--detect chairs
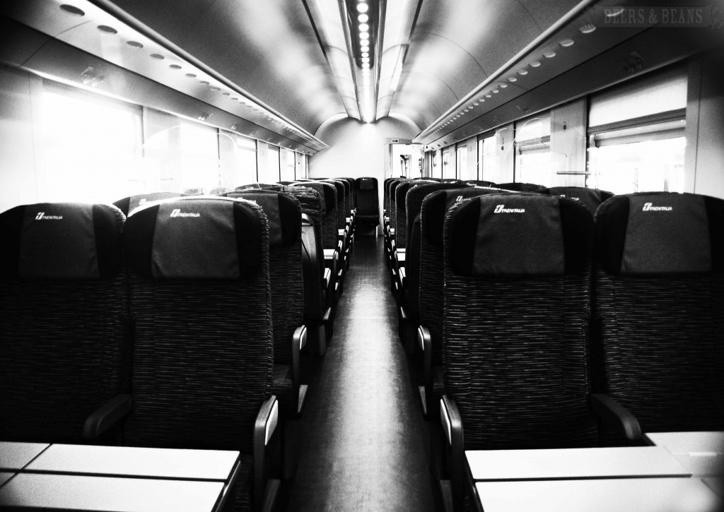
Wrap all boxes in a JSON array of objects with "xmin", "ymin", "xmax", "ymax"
[
  {"xmin": 591, "ymin": 190, "xmax": 724, "ymax": 443},
  {"xmin": 381, "ymin": 176, "xmax": 724, "ymax": 511},
  {"xmin": 433, "ymin": 190, "xmax": 648, "ymax": 511},
  {"xmin": 82, "ymin": 199, "xmax": 284, "ymax": 511},
  {"xmin": 1, "ymin": 202, "xmax": 130, "ymax": 446},
  {"xmin": 0, "ymin": 176, "xmax": 379, "ymax": 512}
]
